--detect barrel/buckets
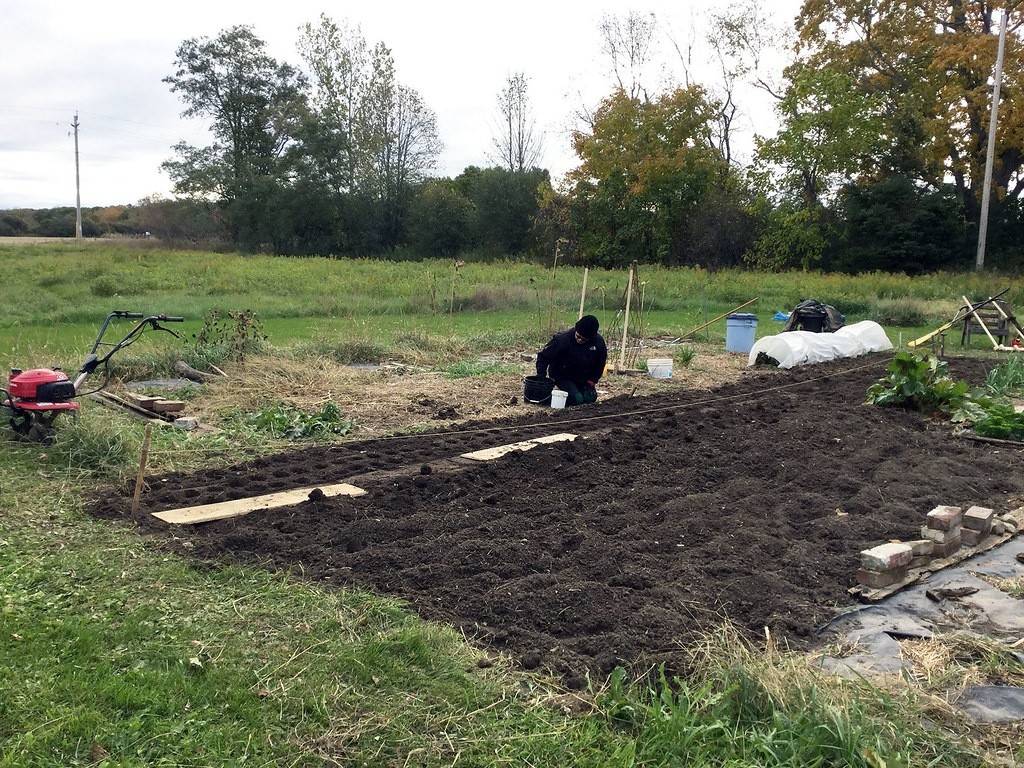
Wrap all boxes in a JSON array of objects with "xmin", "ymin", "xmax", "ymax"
[
  {"xmin": 726, "ymin": 312, "xmax": 759, "ymax": 353},
  {"xmin": 550, "ymin": 390, "xmax": 568, "ymax": 409},
  {"xmin": 521, "ymin": 376, "xmax": 555, "ymax": 407},
  {"xmin": 647, "ymin": 359, "xmax": 673, "ymax": 379}
]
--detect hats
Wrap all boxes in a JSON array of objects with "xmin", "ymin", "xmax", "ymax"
[{"xmin": 575, "ymin": 315, "xmax": 599, "ymax": 338}]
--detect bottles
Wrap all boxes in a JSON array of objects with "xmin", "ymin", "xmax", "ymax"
[{"xmin": 1010, "ymin": 338, "xmax": 1021, "ymax": 348}]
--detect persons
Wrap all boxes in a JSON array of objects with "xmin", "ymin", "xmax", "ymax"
[{"xmin": 536, "ymin": 315, "xmax": 607, "ymax": 406}]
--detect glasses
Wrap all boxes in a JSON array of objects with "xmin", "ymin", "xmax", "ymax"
[{"xmin": 575, "ymin": 333, "xmax": 587, "ymax": 342}]
